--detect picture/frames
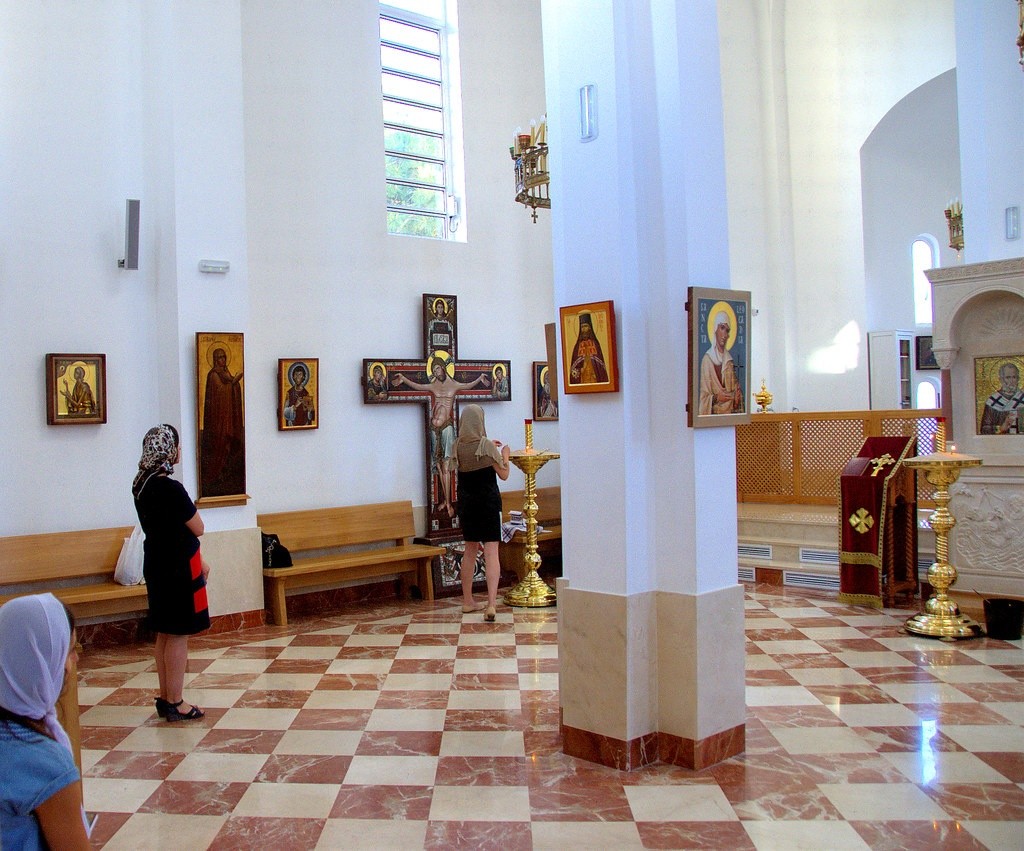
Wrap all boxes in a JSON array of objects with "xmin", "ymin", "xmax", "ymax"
[
  {"xmin": 46, "ymin": 354, "xmax": 107, "ymax": 425},
  {"xmin": 195, "ymin": 332, "xmax": 252, "ymax": 503},
  {"xmin": 916, "ymin": 336, "xmax": 940, "ymax": 370},
  {"xmin": 688, "ymin": 286, "xmax": 751, "ymax": 428},
  {"xmin": 533, "ymin": 361, "xmax": 559, "ymax": 421},
  {"xmin": 278, "ymin": 358, "xmax": 321, "ymax": 431},
  {"xmin": 559, "ymin": 300, "xmax": 619, "ymax": 393}
]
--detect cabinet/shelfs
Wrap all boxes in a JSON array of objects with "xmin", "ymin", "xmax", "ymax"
[{"xmin": 868, "ymin": 329, "xmax": 915, "ymax": 409}]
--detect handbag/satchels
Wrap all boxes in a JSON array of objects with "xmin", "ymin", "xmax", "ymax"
[
  {"xmin": 113, "ymin": 523, "xmax": 146, "ymax": 586},
  {"xmin": 261, "ymin": 531, "xmax": 293, "ymax": 568}
]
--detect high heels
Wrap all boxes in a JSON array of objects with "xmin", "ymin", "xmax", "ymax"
[{"xmin": 165, "ymin": 700, "xmax": 205, "ymax": 722}]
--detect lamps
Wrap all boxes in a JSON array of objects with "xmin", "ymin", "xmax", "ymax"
[{"xmin": 510, "ymin": 112, "xmax": 552, "ymax": 223}]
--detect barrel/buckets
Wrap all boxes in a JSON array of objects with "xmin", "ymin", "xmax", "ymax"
[{"xmin": 982, "ymin": 598, "xmax": 1024, "ymax": 640}]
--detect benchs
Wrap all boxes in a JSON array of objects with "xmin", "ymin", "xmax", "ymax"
[
  {"xmin": 257, "ymin": 501, "xmax": 446, "ymax": 625},
  {"xmin": 498, "ymin": 486, "xmax": 562, "ymax": 583},
  {"xmin": 0, "ymin": 526, "xmax": 149, "ymax": 634}
]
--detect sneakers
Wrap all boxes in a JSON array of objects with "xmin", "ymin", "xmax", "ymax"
[
  {"xmin": 483, "ymin": 606, "xmax": 496, "ymax": 621},
  {"xmin": 462, "ymin": 601, "xmax": 488, "ymax": 612}
]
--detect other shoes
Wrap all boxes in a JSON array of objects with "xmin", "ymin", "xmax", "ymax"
[{"xmin": 154, "ymin": 697, "xmax": 169, "ymax": 718}]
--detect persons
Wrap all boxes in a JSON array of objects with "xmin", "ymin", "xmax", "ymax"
[
  {"xmin": 450, "ymin": 404, "xmax": 511, "ymax": 622},
  {"xmin": 0, "ymin": 591, "xmax": 94, "ymax": 851},
  {"xmin": 132, "ymin": 423, "xmax": 211, "ymax": 722}
]
